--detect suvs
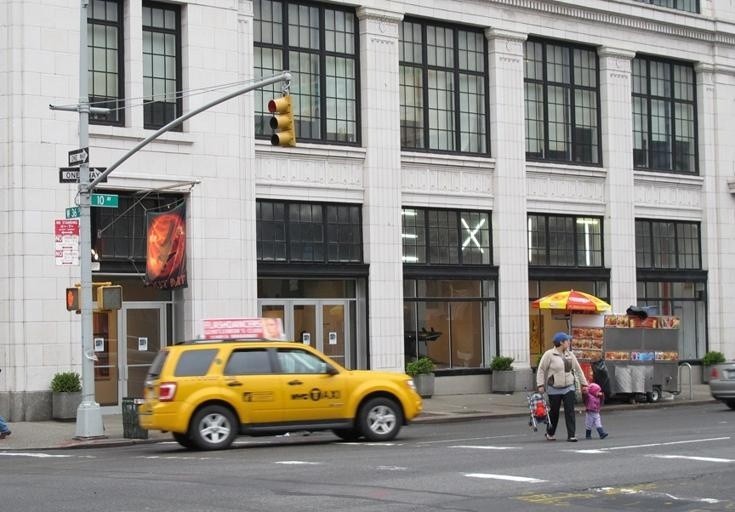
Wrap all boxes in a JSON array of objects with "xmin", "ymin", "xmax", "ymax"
[{"xmin": 137, "ymin": 336, "xmax": 423, "ymax": 452}]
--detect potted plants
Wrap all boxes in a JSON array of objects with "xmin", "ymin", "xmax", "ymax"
[
  {"xmin": 405, "ymin": 356, "xmax": 435, "ymax": 397},
  {"xmin": 702, "ymin": 351, "xmax": 725, "ymax": 382},
  {"xmin": 51, "ymin": 372, "xmax": 83, "ymax": 420},
  {"xmin": 490, "ymin": 354, "xmax": 516, "ymax": 394}
]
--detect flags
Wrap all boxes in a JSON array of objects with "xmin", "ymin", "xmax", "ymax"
[{"xmin": 145, "ymin": 204, "xmax": 189, "ymax": 290}]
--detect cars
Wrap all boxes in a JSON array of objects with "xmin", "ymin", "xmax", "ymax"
[{"xmin": 710, "ymin": 359, "xmax": 735, "ymax": 410}]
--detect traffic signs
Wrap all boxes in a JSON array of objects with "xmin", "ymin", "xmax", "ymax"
[
  {"xmin": 67, "ymin": 148, "xmax": 88, "ymax": 165},
  {"xmin": 59, "ymin": 168, "xmax": 108, "ymax": 183}
]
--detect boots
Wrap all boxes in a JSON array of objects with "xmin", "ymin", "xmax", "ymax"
[
  {"xmin": 597, "ymin": 427, "xmax": 609, "ymax": 439},
  {"xmin": 586, "ymin": 430, "xmax": 592, "ymax": 440}
]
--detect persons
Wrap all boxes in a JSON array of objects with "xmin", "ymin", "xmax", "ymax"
[
  {"xmin": 583, "ymin": 383, "xmax": 609, "ymax": 440},
  {"xmin": 536, "ymin": 332, "xmax": 590, "ymax": 442}
]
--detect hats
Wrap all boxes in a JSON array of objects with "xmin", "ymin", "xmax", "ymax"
[{"xmin": 552, "ymin": 331, "xmax": 573, "ymax": 343}]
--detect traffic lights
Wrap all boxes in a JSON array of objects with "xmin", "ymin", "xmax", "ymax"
[
  {"xmin": 268, "ymin": 96, "xmax": 295, "ymax": 147},
  {"xmin": 97, "ymin": 283, "xmax": 123, "ymax": 312},
  {"xmin": 66, "ymin": 287, "xmax": 80, "ymax": 312}
]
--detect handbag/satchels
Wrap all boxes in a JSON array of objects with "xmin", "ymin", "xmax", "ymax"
[{"xmin": 572, "ymin": 368, "xmax": 583, "ymax": 404}]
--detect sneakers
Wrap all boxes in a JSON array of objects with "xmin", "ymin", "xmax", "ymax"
[
  {"xmin": 545, "ymin": 433, "xmax": 557, "ymax": 440},
  {"xmin": 567, "ymin": 436, "xmax": 577, "ymax": 442}
]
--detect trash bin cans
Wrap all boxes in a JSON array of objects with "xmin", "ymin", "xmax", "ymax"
[{"xmin": 122, "ymin": 397, "xmax": 148, "ymax": 439}]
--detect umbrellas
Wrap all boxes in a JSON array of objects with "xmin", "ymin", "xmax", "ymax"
[{"xmin": 533, "ymin": 288, "xmax": 612, "ymax": 355}]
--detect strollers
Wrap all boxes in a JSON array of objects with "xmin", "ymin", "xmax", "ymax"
[{"xmin": 527, "ymin": 390, "xmax": 554, "ymax": 432}]
